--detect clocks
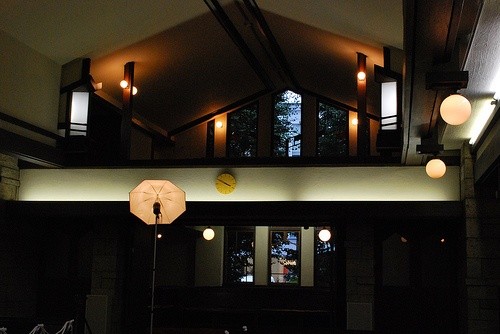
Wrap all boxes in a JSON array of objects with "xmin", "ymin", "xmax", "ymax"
[{"xmin": 214, "ymin": 171, "xmax": 236, "ymax": 195}]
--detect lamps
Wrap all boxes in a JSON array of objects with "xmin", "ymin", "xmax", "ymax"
[
  {"xmin": 423, "ymin": 68, "xmax": 473, "ymax": 126},
  {"xmin": 416, "ymin": 144, "xmax": 447, "ymax": 178}
]
